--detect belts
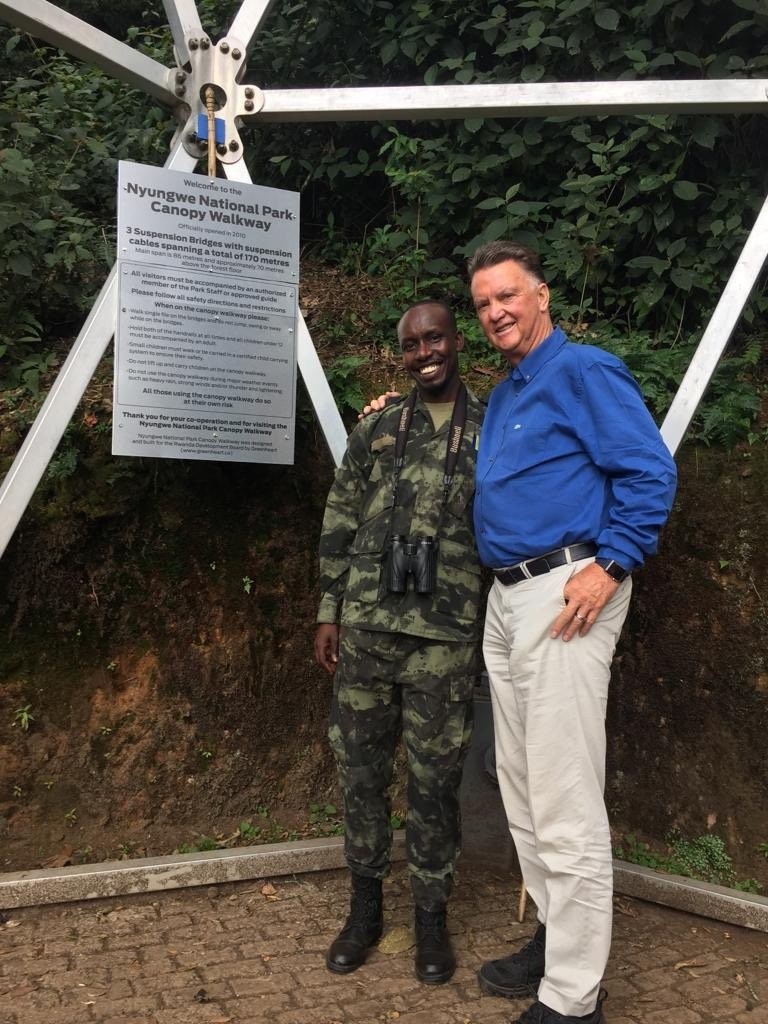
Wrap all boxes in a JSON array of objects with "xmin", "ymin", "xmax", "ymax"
[{"xmin": 488, "ymin": 542, "xmax": 599, "ymax": 585}]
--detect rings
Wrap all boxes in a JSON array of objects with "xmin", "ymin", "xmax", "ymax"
[{"xmin": 573, "ymin": 612, "xmax": 583, "ymax": 622}]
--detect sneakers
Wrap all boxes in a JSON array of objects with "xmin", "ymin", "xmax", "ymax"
[
  {"xmin": 475, "ymin": 923, "xmax": 545, "ymax": 998},
  {"xmin": 510, "ymin": 987, "xmax": 609, "ymax": 1024}
]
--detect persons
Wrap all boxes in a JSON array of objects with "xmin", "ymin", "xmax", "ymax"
[
  {"xmin": 349, "ymin": 233, "xmax": 679, "ymax": 1024},
  {"xmin": 314, "ymin": 293, "xmax": 494, "ymax": 981}
]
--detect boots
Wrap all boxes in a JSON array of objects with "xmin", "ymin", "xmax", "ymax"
[
  {"xmin": 325, "ymin": 896, "xmax": 383, "ymax": 974},
  {"xmin": 410, "ymin": 906, "xmax": 456, "ymax": 985}
]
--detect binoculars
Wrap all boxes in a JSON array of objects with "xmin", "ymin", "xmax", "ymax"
[{"xmin": 389, "ymin": 535, "xmax": 432, "ymax": 596}]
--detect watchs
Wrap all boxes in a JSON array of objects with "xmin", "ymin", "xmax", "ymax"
[{"xmin": 593, "ymin": 558, "xmax": 627, "ymax": 582}]
